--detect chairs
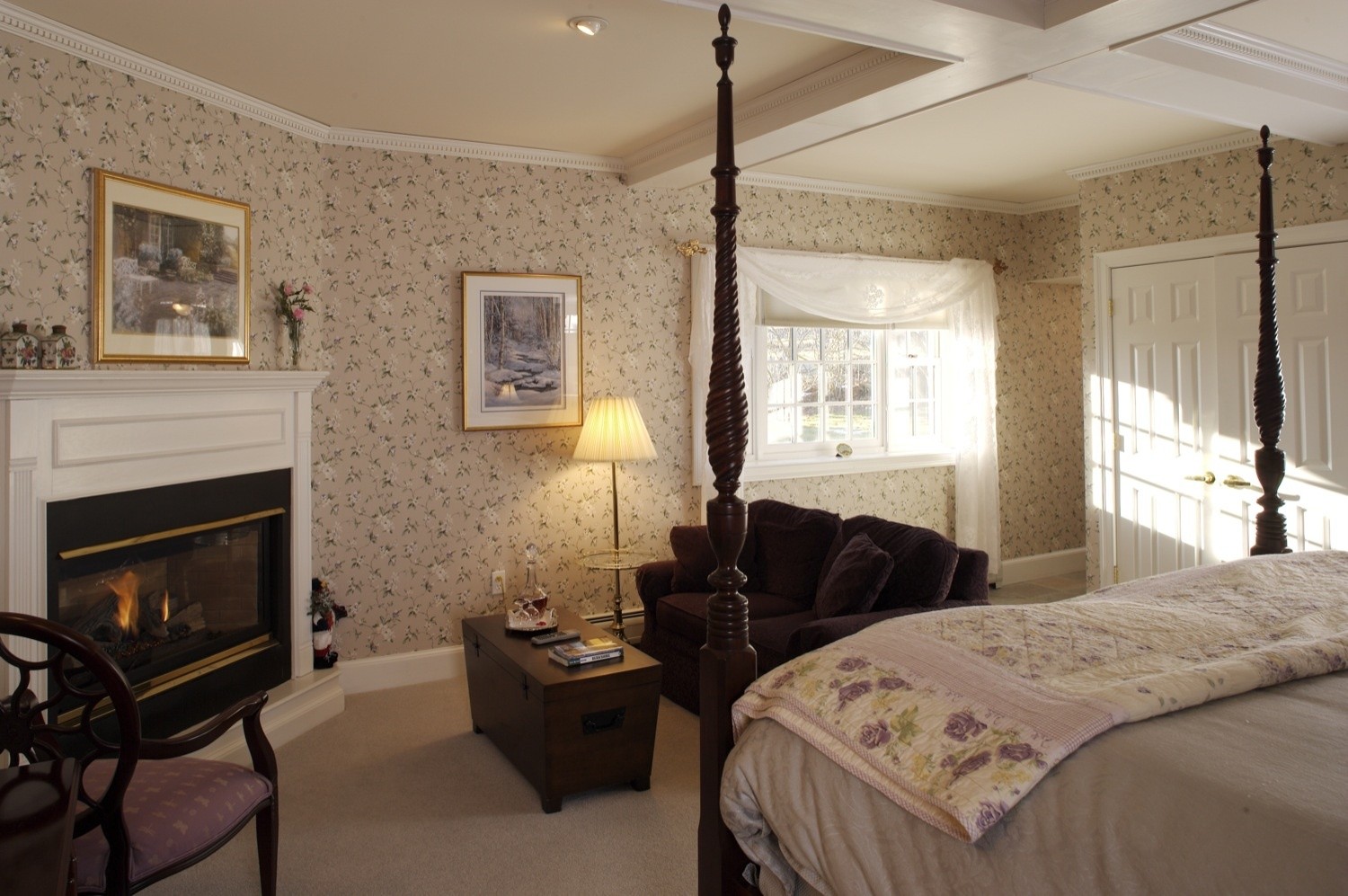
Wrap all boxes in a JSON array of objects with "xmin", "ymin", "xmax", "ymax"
[{"xmin": 1, "ymin": 612, "xmax": 280, "ymax": 896}]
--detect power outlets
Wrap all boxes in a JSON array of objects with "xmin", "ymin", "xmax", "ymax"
[{"xmin": 492, "ymin": 570, "xmax": 506, "ymax": 595}]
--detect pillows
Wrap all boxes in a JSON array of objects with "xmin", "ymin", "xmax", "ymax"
[
  {"xmin": 670, "ymin": 521, "xmax": 760, "ymax": 594},
  {"xmin": 813, "ymin": 532, "xmax": 894, "ymax": 620}
]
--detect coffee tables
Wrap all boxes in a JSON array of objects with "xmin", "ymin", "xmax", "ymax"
[{"xmin": 462, "ymin": 606, "xmax": 663, "ymax": 814}]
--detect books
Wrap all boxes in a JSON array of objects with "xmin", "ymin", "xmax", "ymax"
[{"xmin": 549, "ymin": 636, "xmax": 623, "ymax": 666}]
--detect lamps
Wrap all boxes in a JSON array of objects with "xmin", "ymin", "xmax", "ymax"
[{"xmin": 572, "ymin": 395, "xmax": 658, "ymax": 644}]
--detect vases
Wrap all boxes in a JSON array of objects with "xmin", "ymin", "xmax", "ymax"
[
  {"xmin": 286, "ymin": 323, "xmax": 305, "ymax": 371},
  {"xmin": 313, "ymin": 609, "xmax": 338, "ymax": 671}
]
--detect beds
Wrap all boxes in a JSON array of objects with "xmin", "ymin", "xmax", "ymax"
[{"xmin": 698, "ymin": 551, "xmax": 1348, "ymax": 896}]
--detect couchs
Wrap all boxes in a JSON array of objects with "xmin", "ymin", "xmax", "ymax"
[{"xmin": 636, "ymin": 500, "xmax": 990, "ymax": 718}]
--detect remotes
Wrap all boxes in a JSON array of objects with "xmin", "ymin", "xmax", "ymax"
[{"xmin": 531, "ymin": 629, "xmax": 580, "ymax": 645}]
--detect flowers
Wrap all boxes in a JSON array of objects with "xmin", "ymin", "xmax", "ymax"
[
  {"xmin": 275, "ymin": 280, "xmax": 316, "ymax": 364},
  {"xmin": 307, "ymin": 583, "xmax": 337, "ymax": 632}
]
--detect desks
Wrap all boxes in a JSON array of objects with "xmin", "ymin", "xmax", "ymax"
[{"xmin": 1, "ymin": 758, "xmax": 82, "ymax": 895}]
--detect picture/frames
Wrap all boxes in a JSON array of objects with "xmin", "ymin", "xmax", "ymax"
[
  {"xmin": 91, "ymin": 167, "xmax": 251, "ymax": 365},
  {"xmin": 460, "ymin": 271, "xmax": 583, "ymax": 432}
]
